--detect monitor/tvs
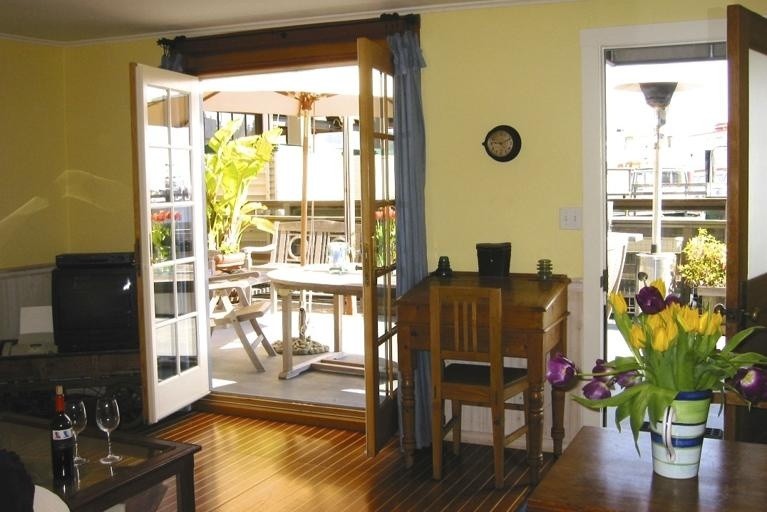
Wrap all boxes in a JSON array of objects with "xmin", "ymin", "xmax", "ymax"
[{"xmin": 50, "ymin": 252, "xmax": 137, "ymax": 354}]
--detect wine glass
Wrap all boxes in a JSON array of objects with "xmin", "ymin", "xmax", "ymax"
[
  {"xmin": 66, "ymin": 401, "xmax": 90, "ymax": 468},
  {"xmin": 96, "ymin": 397, "xmax": 123, "ymax": 464}
]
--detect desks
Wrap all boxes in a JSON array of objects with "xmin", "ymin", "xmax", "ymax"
[
  {"xmin": 266, "ymin": 262, "xmax": 397, "ymax": 381},
  {"xmin": 394, "ymin": 270, "xmax": 571, "ymax": 488},
  {"xmin": 527, "ymin": 425, "xmax": 767, "ymax": 512}
]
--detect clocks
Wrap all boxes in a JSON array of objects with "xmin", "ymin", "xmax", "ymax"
[{"xmin": 481, "ymin": 125, "xmax": 522, "ymax": 162}]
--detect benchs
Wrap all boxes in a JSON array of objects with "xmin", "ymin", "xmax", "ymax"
[{"xmin": 243, "ymin": 219, "xmax": 363, "ymax": 315}]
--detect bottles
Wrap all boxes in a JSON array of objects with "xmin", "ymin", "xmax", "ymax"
[
  {"xmin": 535, "ymin": 259, "xmax": 553, "ymax": 281},
  {"xmin": 50, "ymin": 384, "xmax": 76, "ymax": 481},
  {"xmin": 435, "ymin": 256, "xmax": 452, "ymax": 279}
]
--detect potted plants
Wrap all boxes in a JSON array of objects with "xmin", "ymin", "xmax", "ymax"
[{"xmin": 205, "ymin": 119, "xmax": 284, "ymax": 304}]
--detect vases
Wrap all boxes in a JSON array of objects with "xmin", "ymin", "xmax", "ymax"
[{"xmin": 650, "ymin": 387, "xmax": 712, "ymax": 480}]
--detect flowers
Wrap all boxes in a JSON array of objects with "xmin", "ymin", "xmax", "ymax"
[
  {"xmin": 544, "ymin": 271, "xmax": 767, "ymax": 463},
  {"xmin": 150, "ymin": 210, "xmax": 183, "ymax": 262}
]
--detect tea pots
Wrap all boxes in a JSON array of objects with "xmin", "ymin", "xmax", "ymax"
[{"xmin": 327, "ymin": 237, "xmax": 350, "ymax": 274}]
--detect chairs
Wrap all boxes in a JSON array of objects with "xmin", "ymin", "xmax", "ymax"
[
  {"xmin": 427, "ymin": 283, "xmax": 528, "ymax": 489},
  {"xmin": 208, "ymin": 250, "xmax": 277, "ymax": 373}
]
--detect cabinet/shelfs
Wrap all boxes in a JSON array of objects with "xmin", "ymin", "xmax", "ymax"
[{"xmin": 0, "ymin": 346, "xmax": 147, "ymax": 433}]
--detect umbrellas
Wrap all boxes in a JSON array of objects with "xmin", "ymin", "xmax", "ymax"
[
  {"xmin": 147, "ymin": 94, "xmax": 189, "ymax": 127},
  {"xmin": 202, "ymin": 66, "xmax": 395, "ymax": 341}
]
